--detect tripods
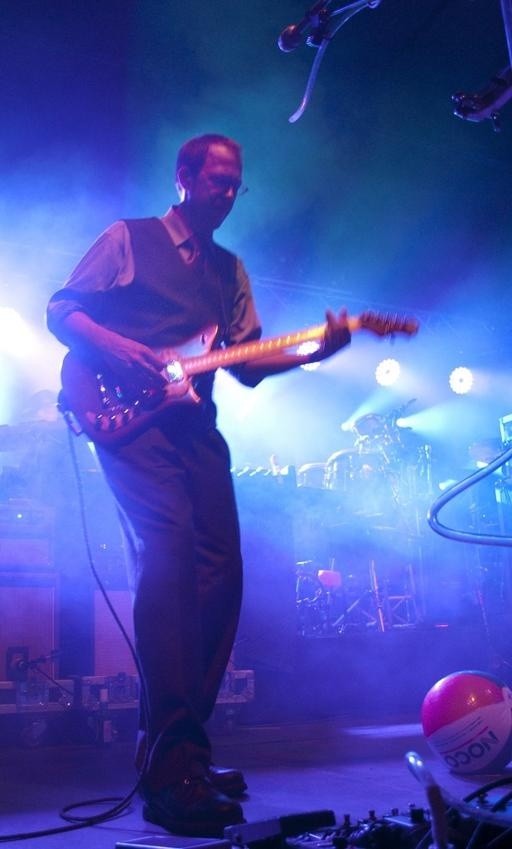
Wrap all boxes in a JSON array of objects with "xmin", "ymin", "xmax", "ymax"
[{"xmin": 295, "ymin": 424, "xmax": 504, "ymax": 632}]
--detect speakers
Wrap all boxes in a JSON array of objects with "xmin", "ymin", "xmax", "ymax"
[{"xmin": 0, "ymin": 469, "xmax": 234, "ymax": 690}]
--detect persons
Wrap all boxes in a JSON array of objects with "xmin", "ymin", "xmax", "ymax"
[{"xmin": 44, "ymin": 132, "xmax": 354, "ymax": 841}]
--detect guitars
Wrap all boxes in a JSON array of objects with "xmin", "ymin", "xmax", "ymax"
[{"xmin": 60, "ymin": 310, "xmax": 420, "ymax": 444}]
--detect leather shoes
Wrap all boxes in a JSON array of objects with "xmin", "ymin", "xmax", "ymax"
[
  {"xmin": 132, "ymin": 735, "xmax": 246, "ymax": 801},
  {"xmin": 138, "ymin": 784, "xmax": 248, "ymax": 834}
]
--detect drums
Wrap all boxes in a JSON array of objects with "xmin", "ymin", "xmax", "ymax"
[
  {"xmin": 352, "ymin": 414, "xmax": 393, "ymax": 453},
  {"xmin": 296, "ymin": 462, "xmax": 327, "ymax": 490},
  {"xmin": 321, "ymin": 451, "xmax": 364, "ymax": 491}
]
